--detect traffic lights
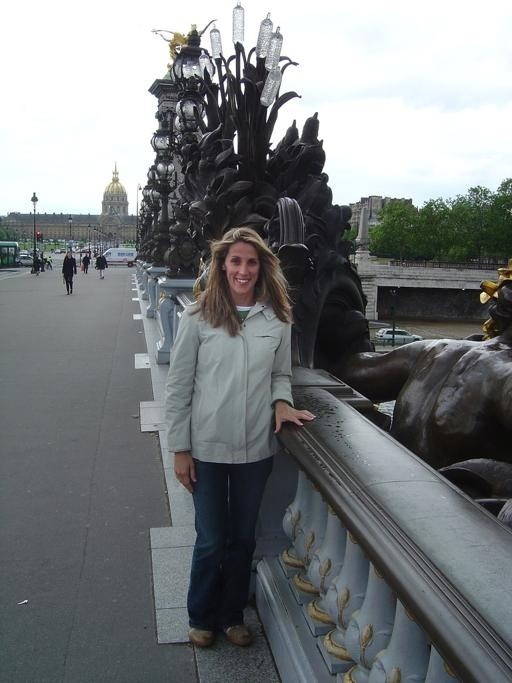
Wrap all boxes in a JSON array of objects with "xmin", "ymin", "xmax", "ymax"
[{"xmin": 36, "ymin": 232, "xmax": 45, "ymax": 244}]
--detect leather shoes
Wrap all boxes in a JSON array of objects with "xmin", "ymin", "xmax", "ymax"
[
  {"xmin": 222, "ymin": 625, "xmax": 251, "ymax": 647},
  {"xmin": 189, "ymin": 627, "xmax": 215, "ymax": 646}
]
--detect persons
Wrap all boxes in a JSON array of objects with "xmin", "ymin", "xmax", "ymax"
[
  {"xmin": 163, "ymin": 226, "xmax": 319, "ymax": 651},
  {"xmin": 81, "ymin": 253, "xmax": 90, "ymax": 275},
  {"xmin": 46, "ymin": 256, "xmax": 54, "ymax": 271},
  {"xmin": 61, "ymin": 251, "xmax": 77, "ymax": 296},
  {"xmin": 96, "ymin": 253, "xmax": 108, "ymax": 280},
  {"xmin": 33, "ymin": 256, "xmax": 41, "ymax": 275}
]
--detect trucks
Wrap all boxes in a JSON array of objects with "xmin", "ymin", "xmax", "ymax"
[{"xmin": 96, "ymin": 247, "xmax": 139, "ymax": 266}]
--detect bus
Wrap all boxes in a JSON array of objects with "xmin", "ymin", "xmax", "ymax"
[{"xmin": 0, "ymin": 241, "xmax": 20, "ymax": 270}]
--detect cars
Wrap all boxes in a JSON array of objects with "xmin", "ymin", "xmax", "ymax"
[
  {"xmin": 15, "ymin": 247, "xmax": 47, "ymax": 268},
  {"xmin": 18, "ymin": 237, "xmax": 74, "ymax": 244},
  {"xmin": 462, "ymin": 333, "xmax": 487, "ymax": 344},
  {"xmin": 52, "ymin": 243, "xmax": 106, "ymax": 255}
]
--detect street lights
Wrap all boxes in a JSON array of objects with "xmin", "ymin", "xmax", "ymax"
[
  {"xmin": 87, "ymin": 224, "xmax": 120, "ymax": 259},
  {"xmin": 30, "ymin": 191, "xmax": 39, "ymax": 275},
  {"xmin": 137, "ymin": 183, "xmax": 145, "ymax": 255},
  {"xmin": 67, "ymin": 213, "xmax": 74, "ymax": 260}
]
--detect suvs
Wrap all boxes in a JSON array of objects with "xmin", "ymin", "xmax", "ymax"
[{"xmin": 375, "ymin": 327, "xmax": 424, "ymax": 346}]
